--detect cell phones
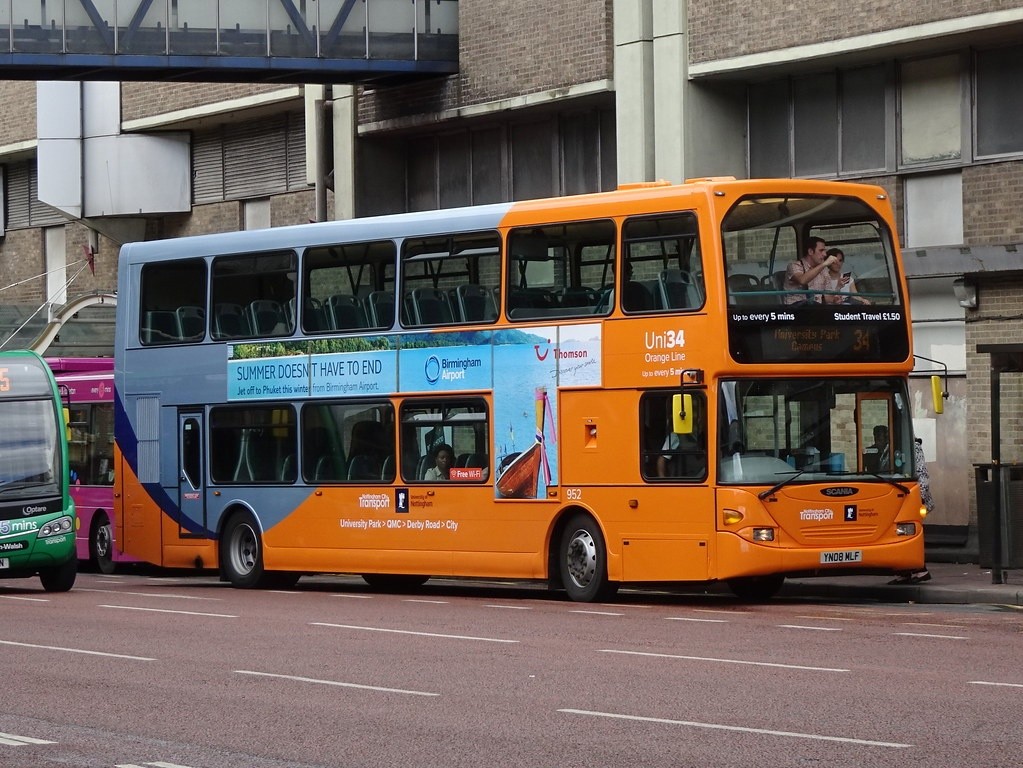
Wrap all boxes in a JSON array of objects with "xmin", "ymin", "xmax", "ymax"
[{"xmin": 843, "ymin": 272, "xmax": 851, "ymax": 283}]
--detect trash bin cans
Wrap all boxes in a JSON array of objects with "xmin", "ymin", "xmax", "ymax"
[{"xmin": 972, "ymin": 463, "xmax": 1023, "ymax": 569}]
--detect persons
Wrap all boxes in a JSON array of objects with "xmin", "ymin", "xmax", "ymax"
[
  {"xmin": 657, "ymin": 412, "xmax": 705, "ymax": 478},
  {"xmin": 861, "ymin": 425, "xmax": 934, "ymax": 585},
  {"xmin": 424, "ymin": 444, "xmax": 455, "ymax": 481},
  {"xmin": 600, "ymin": 259, "xmax": 633, "ymax": 313},
  {"xmin": 783, "ymin": 237, "xmax": 871, "ymax": 307}
]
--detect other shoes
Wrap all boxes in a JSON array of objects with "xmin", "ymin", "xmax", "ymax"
[
  {"xmin": 910, "ymin": 573, "xmax": 932, "ymax": 584},
  {"xmin": 886, "ymin": 579, "xmax": 909, "ymax": 584}
]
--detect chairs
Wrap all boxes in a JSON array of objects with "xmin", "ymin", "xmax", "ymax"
[
  {"xmin": 217, "ymin": 426, "xmax": 486, "ymax": 482},
  {"xmin": 146, "ymin": 269, "xmax": 790, "ymax": 343}
]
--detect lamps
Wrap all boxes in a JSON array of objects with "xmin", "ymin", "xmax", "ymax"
[{"xmin": 952, "ymin": 278, "xmax": 976, "ymax": 309}]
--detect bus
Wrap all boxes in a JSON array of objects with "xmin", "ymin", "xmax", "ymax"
[
  {"xmin": 111, "ymin": 173, "xmax": 950, "ymax": 602},
  {"xmin": 0, "ymin": 349, "xmax": 142, "ymax": 593}
]
